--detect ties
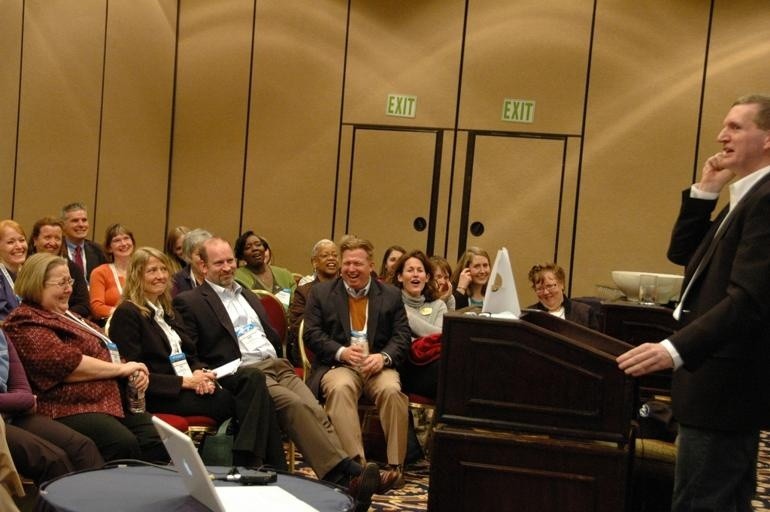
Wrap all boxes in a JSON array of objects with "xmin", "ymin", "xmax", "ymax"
[{"xmin": 75, "ymin": 245, "xmax": 84, "ymax": 279}]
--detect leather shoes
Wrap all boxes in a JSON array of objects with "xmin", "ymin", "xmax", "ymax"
[
  {"xmin": 348, "ymin": 462, "xmax": 380, "ymax": 512},
  {"xmin": 349, "ymin": 468, "xmax": 398, "ymax": 494},
  {"xmin": 393, "ymin": 472, "xmax": 405, "ymax": 489}
]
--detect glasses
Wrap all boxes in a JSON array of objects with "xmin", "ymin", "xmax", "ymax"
[{"xmin": 43, "ymin": 279, "xmax": 74, "ymax": 286}]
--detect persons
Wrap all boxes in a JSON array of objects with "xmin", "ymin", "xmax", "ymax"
[
  {"xmin": 453, "ymin": 247, "xmax": 491, "ymax": 312},
  {"xmin": 0, "ymin": 324, "xmax": 108, "ymax": 486},
  {"xmin": 615, "ymin": 95, "xmax": 770, "ymax": 511},
  {"xmin": 172, "ymin": 237, "xmax": 402, "ymax": 510},
  {"xmin": 389, "ymin": 249, "xmax": 448, "ymax": 397},
  {"xmin": 289, "ymin": 240, "xmax": 342, "ymax": 369},
  {"xmin": 58, "ymin": 204, "xmax": 109, "ymax": 290},
  {"xmin": 5, "ymin": 254, "xmax": 171, "ymax": 468},
  {"xmin": 29, "ymin": 218, "xmax": 90, "ymax": 318},
  {"xmin": 524, "ymin": 264, "xmax": 603, "ymax": 335},
  {"xmin": 231, "ymin": 231, "xmax": 296, "ymax": 313},
  {"xmin": 88, "ymin": 223, "xmax": 138, "ymax": 320},
  {"xmin": 161, "ymin": 226, "xmax": 189, "ymax": 273},
  {"xmin": 170, "ymin": 228, "xmax": 211, "ymax": 295},
  {"xmin": 428, "ymin": 254, "xmax": 456, "ymax": 318},
  {"xmin": 107, "ymin": 247, "xmax": 279, "ymax": 485},
  {"xmin": 0, "ymin": 218, "xmax": 30, "ymax": 321},
  {"xmin": 378, "ymin": 246, "xmax": 407, "ymax": 287},
  {"xmin": 0, "ymin": 414, "xmax": 55, "ymax": 512},
  {"xmin": 306, "ymin": 236, "xmax": 411, "ymax": 494}
]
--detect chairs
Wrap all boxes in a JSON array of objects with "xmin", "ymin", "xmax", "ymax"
[
  {"xmin": 106, "ymin": 314, "xmax": 216, "ymax": 435},
  {"xmin": 405, "ymin": 393, "xmax": 439, "ymax": 458},
  {"xmin": 254, "ymin": 289, "xmax": 297, "ymax": 473},
  {"xmin": 299, "ymin": 321, "xmax": 378, "ymax": 436}
]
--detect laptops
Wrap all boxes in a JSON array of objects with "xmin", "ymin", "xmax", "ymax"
[{"xmin": 151, "ymin": 413, "xmax": 318, "ymax": 512}]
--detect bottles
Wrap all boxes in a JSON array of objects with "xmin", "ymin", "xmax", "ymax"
[
  {"xmin": 126, "ymin": 371, "xmax": 146, "ymax": 413},
  {"xmin": 352, "ymin": 333, "xmax": 368, "ymax": 359}
]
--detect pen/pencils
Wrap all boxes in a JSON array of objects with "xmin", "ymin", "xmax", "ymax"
[{"xmin": 202, "ymin": 368, "xmax": 223, "ymax": 390}]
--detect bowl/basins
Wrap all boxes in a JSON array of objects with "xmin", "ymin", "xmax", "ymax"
[{"xmin": 610, "ymin": 271, "xmax": 685, "ymax": 304}]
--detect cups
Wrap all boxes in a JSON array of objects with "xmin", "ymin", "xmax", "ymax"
[{"xmin": 638, "ymin": 274, "xmax": 658, "ymax": 306}]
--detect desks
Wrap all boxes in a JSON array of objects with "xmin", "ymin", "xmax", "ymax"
[
  {"xmin": 427, "ymin": 305, "xmax": 637, "ymax": 512},
  {"xmin": 39, "ymin": 465, "xmax": 356, "ymax": 512}
]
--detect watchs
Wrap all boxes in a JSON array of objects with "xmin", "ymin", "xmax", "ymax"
[{"xmin": 382, "ymin": 354, "xmax": 390, "ymax": 365}]
